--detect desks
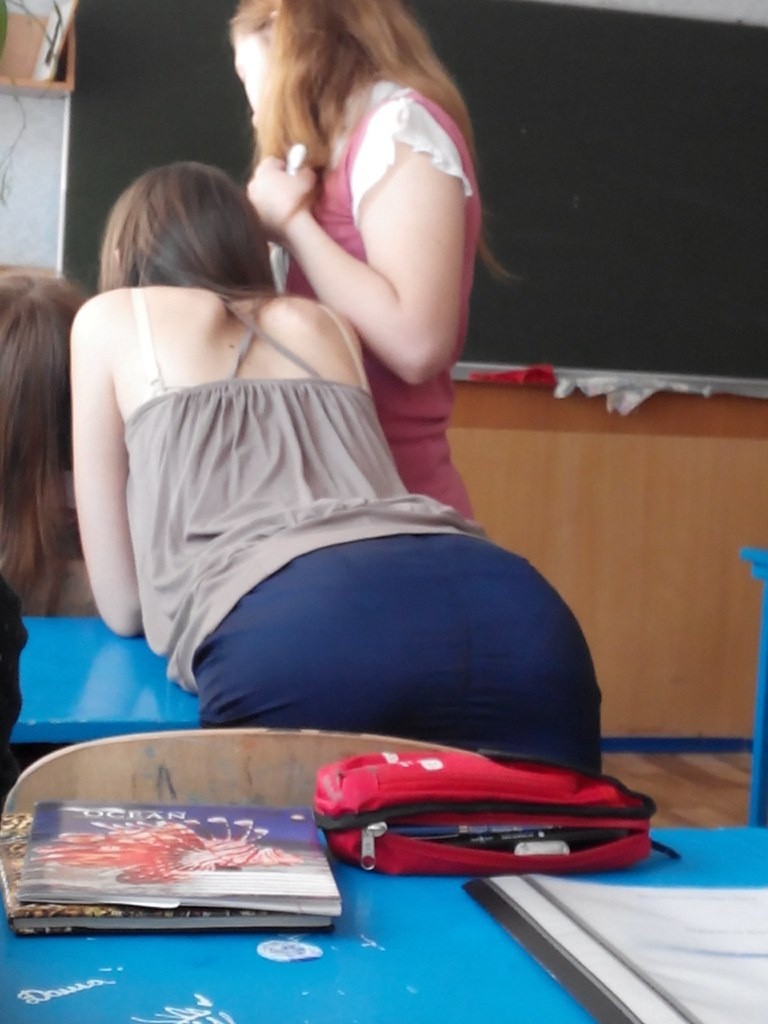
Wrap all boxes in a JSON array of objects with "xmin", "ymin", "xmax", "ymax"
[
  {"xmin": 0, "ymin": 827, "xmax": 768, "ymax": 1024},
  {"xmin": 9, "ymin": 616, "xmax": 202, "ymax": 744}
]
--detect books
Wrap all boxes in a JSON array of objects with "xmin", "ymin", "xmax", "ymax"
[{"xmin": 0, "ymin": 800, "xmax": 343, "ymax": 937}]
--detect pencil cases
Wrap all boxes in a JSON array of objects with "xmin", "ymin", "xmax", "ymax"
[{"xmin": 312, "ymin": 751, "xmax": 679, "ymax": 876}]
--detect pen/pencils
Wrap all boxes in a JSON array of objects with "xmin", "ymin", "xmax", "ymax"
[{"xmin": 388, "ymin": 824, "xmax": 635, "ymax": 851}]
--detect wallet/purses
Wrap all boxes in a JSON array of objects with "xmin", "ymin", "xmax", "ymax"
[{"xmin": 312, "ymin": 752, "xmax": 681, "ymax": 874}]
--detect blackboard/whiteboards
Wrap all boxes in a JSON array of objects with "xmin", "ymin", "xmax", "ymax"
[{"xmin": 57, "ymin": 0, "xmax": 768, "ymax": 396}]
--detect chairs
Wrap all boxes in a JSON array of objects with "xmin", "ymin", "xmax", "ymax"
[{"xmin": 3, "ymin": 727, "xmax": 484, "ymax": 823}]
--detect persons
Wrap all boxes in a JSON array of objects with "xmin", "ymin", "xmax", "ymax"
[
  {"xmin": 227, "ymin": 0, "xmax": 481, "ymax": 522},
  {"xmin": 0, "ymin": 263, "xmax": 87, "ymax": 806},
  {"xmin": 69, "ymin": 161, "xmax": 603, "ymax": 778}
]
